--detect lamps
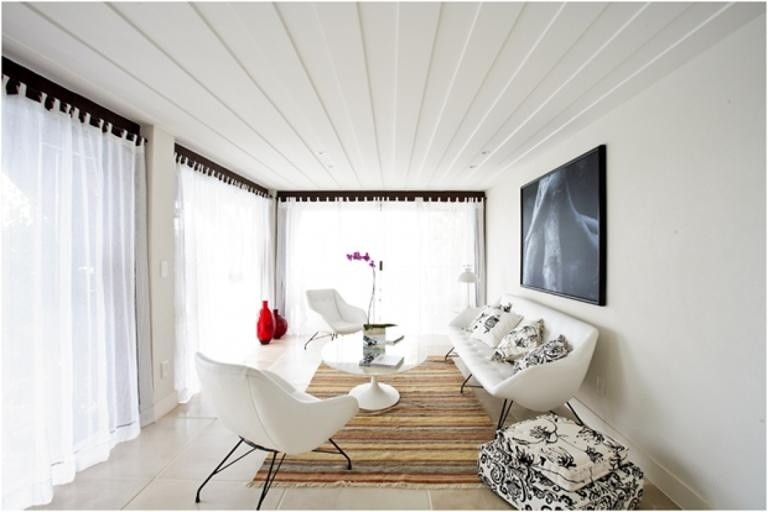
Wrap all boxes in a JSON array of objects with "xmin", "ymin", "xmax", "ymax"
[{"xmin": 458, "ymin": 264, "xmax": 476, "ymax": 305}]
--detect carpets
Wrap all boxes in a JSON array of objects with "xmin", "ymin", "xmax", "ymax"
[{"xmin": 245, "ymin": 355, "xmax": 500, "ymax": 489}]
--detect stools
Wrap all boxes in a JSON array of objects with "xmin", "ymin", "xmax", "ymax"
[{"xmin": 477, "ymin": 413, "xmax": 645, "ymax": 511}]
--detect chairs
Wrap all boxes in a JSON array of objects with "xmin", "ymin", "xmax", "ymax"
[
  {"xmin": 194, "ymin": 353, "xmax": 360, "ymax": 511},
  {"xmin": 303, "ymin": 288, "xmax": 368, "ymax": 351}
]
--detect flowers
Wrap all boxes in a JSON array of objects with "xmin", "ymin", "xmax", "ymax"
[{"xmin": 346, "ymin": 250, "xmax": 383, "ymax": 325}]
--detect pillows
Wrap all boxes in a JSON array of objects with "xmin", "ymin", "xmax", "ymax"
[
  {"xmin": 462, "ymin": 302, "xmax": 512, "ymax": 332},
  {"xmin": 495, "ymin": 414, "xmax": 629, "ymax": 491},
  {"xmin": 513, "ymin": 334, "xmax": 569, "ymax": 376},
  {"xmin": 471, "ymin": 308, "xmax": 523, "ymax": 348},
  {"xmin": 488, "ymin": 318, "xmax": 543, "ymax": 363}
]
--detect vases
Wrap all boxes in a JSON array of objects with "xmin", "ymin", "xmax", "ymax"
[
  {"xmin": 256, "ymin": 301, "xmax": 276, "ymax": 345},
  {"xmin": 272, "ymin": 308, "xmax": 288, "ymax": 339}
]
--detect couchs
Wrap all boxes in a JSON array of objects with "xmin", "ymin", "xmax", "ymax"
[{"xmin": 444, "ymin": 294, "xmax": 599, "ymax": 439}]
